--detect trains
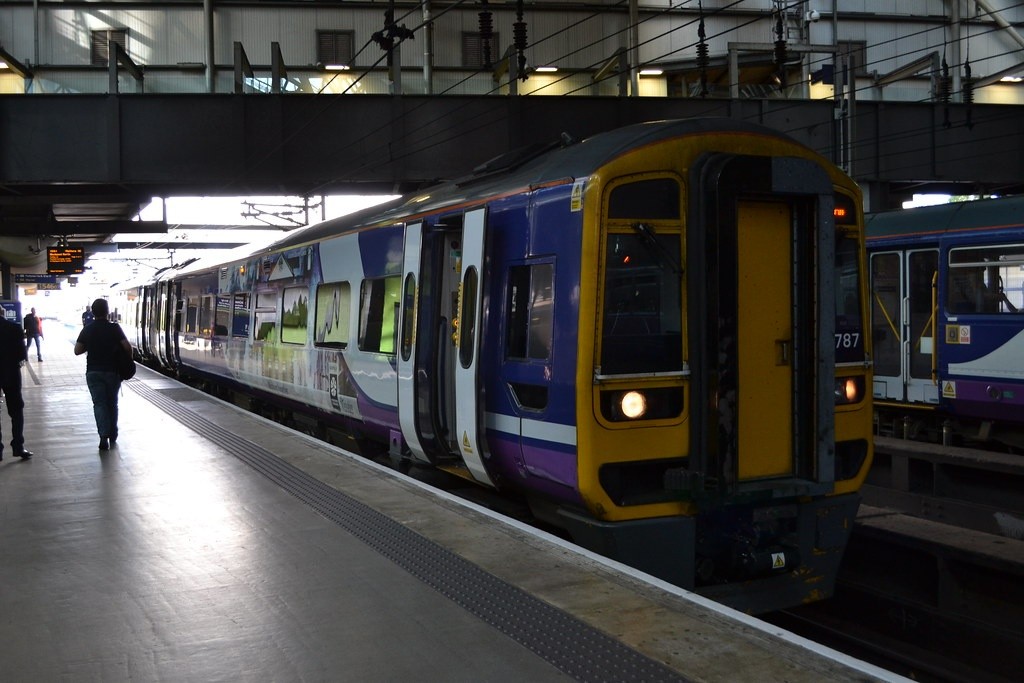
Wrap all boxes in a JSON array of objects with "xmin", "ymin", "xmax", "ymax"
[
  {"xmin": 862, "ymin": 195, "xmax": 1023, "ymax": 441},
  {"xmin": 93, "ymin": 111, "xmax": 875, "ymax": 619}
]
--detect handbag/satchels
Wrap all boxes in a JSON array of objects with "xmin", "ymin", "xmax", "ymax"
[{"xmin": 113, "ymin": 339, "xmax": 136, "ymax": 380}]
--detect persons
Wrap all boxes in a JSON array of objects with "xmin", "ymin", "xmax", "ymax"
[
  {"xmin": 74, "ymin": 298, "xmax": 132, "ymax": 449},
  {"xmin": 0, "ymin": 303, "xmax": 34, "ymax": 463},
  {"xmin": 82, "ymin": 306, "xmax": 95, "ymax": 328},
  {"xmin": 23, "ymin": 308, "xmax": 43, "ymax": 362}
]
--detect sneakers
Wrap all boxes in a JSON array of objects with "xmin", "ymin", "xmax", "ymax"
[
  {"xmin": 98, "ymin": 437, "xmax": 108, "ymax": 449},
  {"xmin": 109, "ymin": 436, "xmax": 118, "ymax": 443}
]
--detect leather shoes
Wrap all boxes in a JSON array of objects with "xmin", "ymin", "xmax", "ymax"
[
  {"xmin": 12, "ymin": 446, "xmax": 34, "ymax": 459},
  {"xmin": 0, "ymin": 450, "xmax": 3, "ymax": 461}
]
